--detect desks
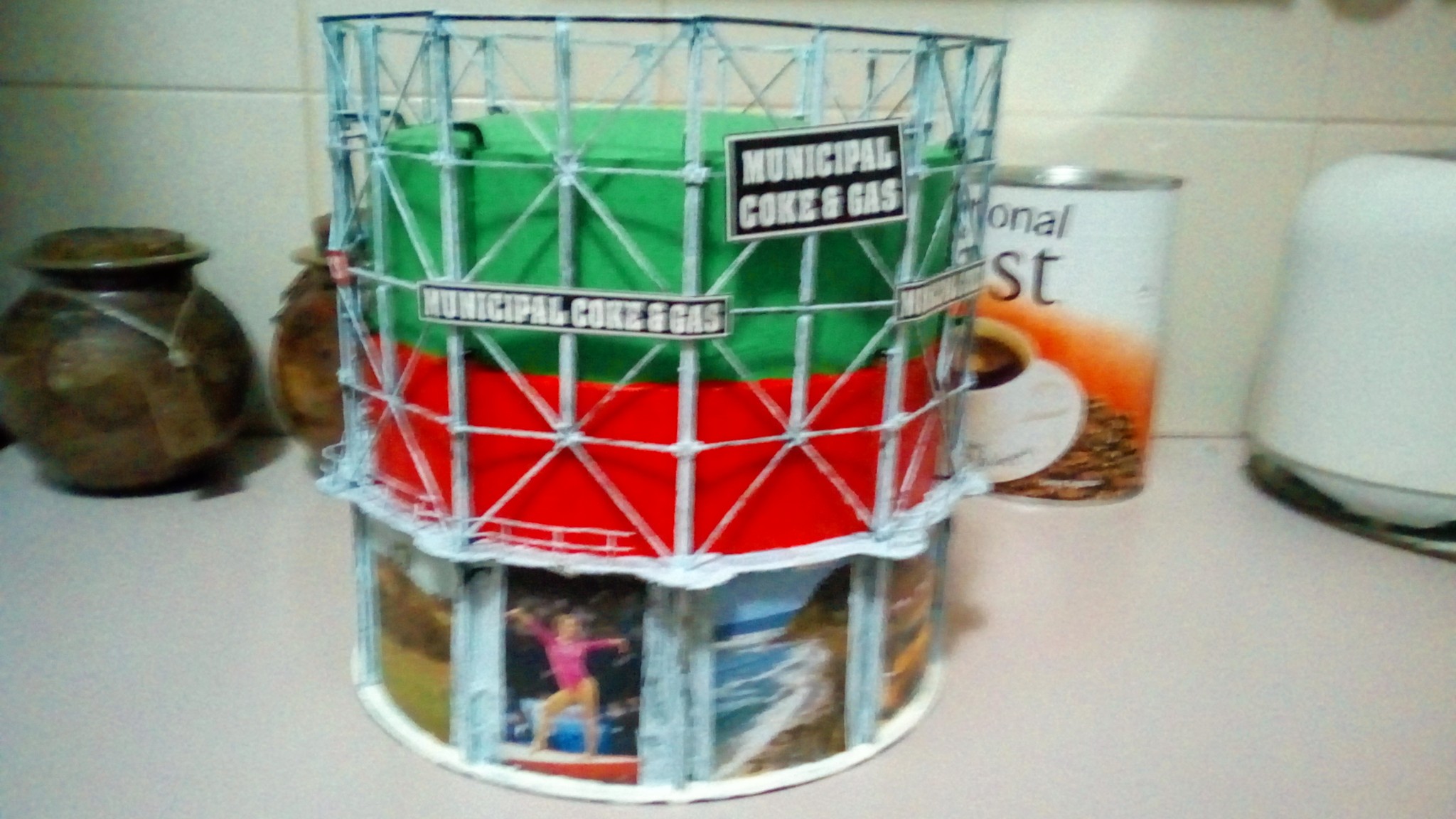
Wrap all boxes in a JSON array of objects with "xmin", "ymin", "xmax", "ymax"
[{"xmin": 0, "ymin": 437, "xmax": 1456, "ymax": 818}]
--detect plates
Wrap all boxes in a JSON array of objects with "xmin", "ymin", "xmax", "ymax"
[{"xmin": 961, "ymin": 356, "xmax": 1086, "ymax": 483}]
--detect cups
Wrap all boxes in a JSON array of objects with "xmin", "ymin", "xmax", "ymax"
[{"xmin": 962, "ymin": 320, "xmax": 1033, "ymax": 446}]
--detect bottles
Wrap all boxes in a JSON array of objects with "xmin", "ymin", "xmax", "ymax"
[
  {"xmin": 0, "ymin": 226, "xmax": 253, "ymax": 498},
  {"xmin": 270, "ymin": 210, "xmax": 372, "ymax": 458}
]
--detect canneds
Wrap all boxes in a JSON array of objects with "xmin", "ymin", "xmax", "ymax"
[{"xmin": 958, "ymin": 162, "xmax": 1183, "ymax": 508}]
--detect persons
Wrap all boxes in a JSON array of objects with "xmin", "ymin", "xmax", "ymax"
[{"xmin": 505, "ymin": 605, "xmax": 629, "ymax": 756}]
